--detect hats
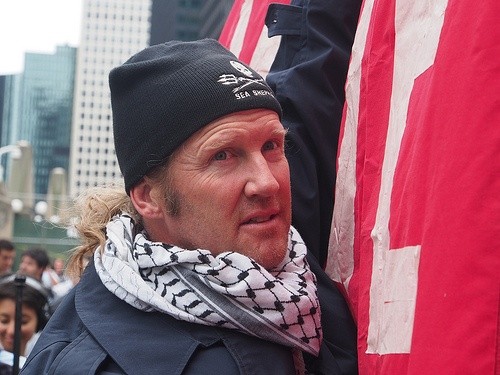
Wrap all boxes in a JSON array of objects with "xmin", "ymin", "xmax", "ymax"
[{"xmin": 108, "ymin": 37, "xmax": 284, "ymax": 191}]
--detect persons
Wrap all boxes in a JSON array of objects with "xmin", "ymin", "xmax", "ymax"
[
  {"xmin": 22, "ymin": 0, "xmax": 361, "ymax": 375},
  {"xmin": 0, "ymin": 274, "xmax": 54, "ymax": 375},
  {"xmin": 0, "ymin": 238, "xmax": 94, "ymax": 302}
]
